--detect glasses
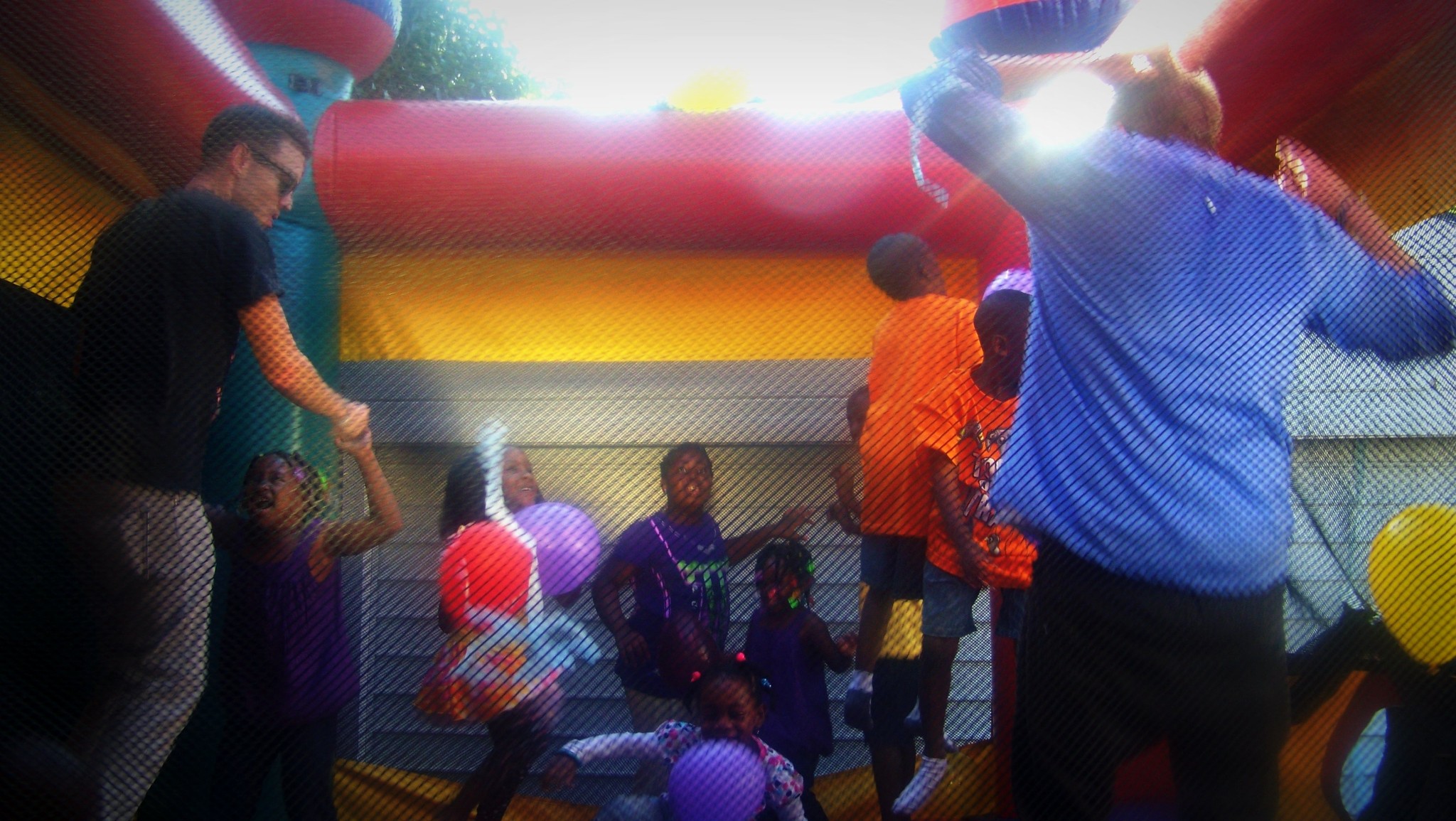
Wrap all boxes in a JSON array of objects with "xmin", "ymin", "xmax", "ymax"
[{"xmin": 250, "ymin": 147, "xmax": 296, "ymax": 195}]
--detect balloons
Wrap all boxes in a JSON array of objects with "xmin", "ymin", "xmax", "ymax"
[
  {"xmin": 434, "ymin": 524, "xmax": 533, "ymax": 633},
  {"xmin": 1369, "ymin": 502, "xmax": 1455, "ymax": 667},
  {"xmin": 507, "ymin": 502, "xmax": 599, "ymax": 595},
  {"xmin": 666, "ymin": 737, "xmax": 767, "ymax": 821}
]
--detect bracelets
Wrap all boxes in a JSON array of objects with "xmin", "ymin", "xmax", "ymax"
[{"xmin": 1334, "ymin": 188, "xmax": 1369, "ymax": 229}]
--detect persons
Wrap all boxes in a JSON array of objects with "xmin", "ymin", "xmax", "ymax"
[
  {"xmin": 401, "ymin": 236, "xmax": 1034, "ymax": 821},
  {"xmin": 0, "ymin": 103, "xmax": 400, "ymax": 821},
  {"xmin": 904, "ymin": 53, "xmax": 1456, "ymax": 821}
]
